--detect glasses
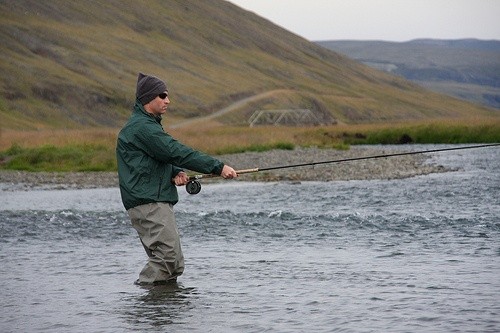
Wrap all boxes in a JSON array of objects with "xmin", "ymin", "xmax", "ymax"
[{"xmin": 159, "ymin": 93, "xmax": 168, "ymax": 99}]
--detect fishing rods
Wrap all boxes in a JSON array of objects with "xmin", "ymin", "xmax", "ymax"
[{"xmin": 170, "ymin": 142, "xmax": 500, "ymax": 195}]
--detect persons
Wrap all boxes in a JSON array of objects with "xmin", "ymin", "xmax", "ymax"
[{"xmin": 116, "ymin": 72, "xmax": 238, "ymax": 285}]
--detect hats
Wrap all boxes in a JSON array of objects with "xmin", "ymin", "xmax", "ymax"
[{"xmin": 137, "ymin": 72, "xmax": 167, "ymax": 105}]
